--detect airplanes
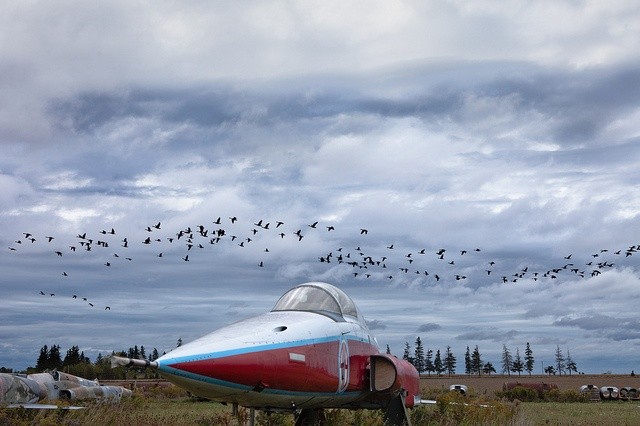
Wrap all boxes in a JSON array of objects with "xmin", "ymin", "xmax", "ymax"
[
  {"xmin": 111, "ymin": 281, "xmax": 496, "ymax": 426},
  {"xmin": 0, "ymin": 368, "xmax": 132, "ymax": 410}
]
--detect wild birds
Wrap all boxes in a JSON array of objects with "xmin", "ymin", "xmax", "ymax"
[
  {"xmin": 105, "ymin": 242, "xmax": 109, "ymax": 247},
  {"xmin": 319, "ymin": 252, "xmax": 333, "ymax": 263},
  {"xmin": 146, "ymin": 227, "xmax": 153, "ymax": 232},
  {"xmin": 183, "ymin": 255, "xmax": 190, "ymax": 261},
  {"xmin": 522, "ymin": 267, "xmax": 529, "ymax": 272},
  {"xmin": 407, "ymin": 253, "xmax": 412, "ymax": 257},
  {"xmin": 593, "ymin": 254, "xmax": 599, "ymax": 258},
  {"xmin": 114, "ymin": 253, "xmax": 120, "ymax": 257},
  {"xmin": 209, "ymin": 239, "xmax": 216, "ymax": 244},
  {"xmin": 581, "ymin": 271, "xmax": 586, "ymax": 273},
  {"xmin": 359, "ymin": 253, "xmax": 365, "ymax": 256},
  {"xmin": 185, "ymin": 227, "xmax": 191, "ymax": 234},
  {"xmin": 96, "ymin": 241, "xmax": 101, "ymax": 245},
  {"xmin": 337, "ymin": 248, "xmax": 343, "ymax": 251},
  {"xmin": 294, "ymin": 229, "xmax": 302, "ymax": 236},
  {"xmin": 595, "ymin": 270, "xmax": 602, "ymax": 276},
  {"xmin": 533, "ymin": 272, "xmax": 540, "ymax": 276},
  {"xmin": 41, "ymin": 291, "xmax": 46, "ymax": 295},
  {"xmin": 101, "ymin": 230, "xmax": 107, "ymax": 234},
  {"xmin": 562, "ymin": 265, "xmax": 568, "ymax": 269},
  {"xmin": 101, "ymin": 241, "xmax": 106, "ymax": 247},
  {"xmin": 565, "ymin": 254, "xmax": 573, "ymax": 259},
  {"xmin": 252, "ymin": 228, "xmax": 259, "ymax": 235},
  {"xmin": 354, "ymin": 273, "xmax": 359, "ymax": 277},
  {"xmin": 361, "ymin": 229, "xmax": 369, "ymax": 234},
  {"xmin": 88, "ymin": 239, "xmax": 94, "ymax": 243},
  {"xmin": 85, "ymin": 247, "xmax": 92, "ymax": 251},
  {"xmin": 415, "ymin": 270, "xmax": 421, "ymax": 274},
  {"xmin": 450, "ymin": 260, "xmax": 455, "ymax": 264},
  {"xmin": 143, "ymin": 239, "xmax": 151, "ymax": 244},
  {"xmin": 30, "ymin": 238, "xmax": 37, "ymax": 243},
  {"xmin": 405, "ymin": 268, "xmax": 409, "ymax": 273},
  {"xmin": 265, "ymin": 248, "xmax": 270, "ymax": 252},
  {"xmin": 490, "ymin": 261, "xmax": 496, "ymax": 266},
  {"xmin": 504, "ymin": 276, "xmax": 508, "ymax": 283},
  {"xmin": 108, "ymin": 228, "xmax": 116, "ymax": 234},
  {"xmin": 572, "ymin": 268, "xmax": 579, "ymax": 273},
  {"xmin": 628, "ymin": 248, "xmax": 638, "ymax": 252},
  {"xmin": 616, "ymin": 250, "xmax": 622, "ymax": 254},
  {"xmin": 424, "ymin": 271, "xmax": 430, "ymax": 276},
  {"xmin": 198, "ymin": 225, "xmax": 204, "ymax": 231},
  {"xmin": 382, "ymin": 257, "xmax": 388, "ymax": 261},
  {"xmin": 126, "ymin": 257, "xmax": 133, "ymax": 261},
  {"xmin": 106, "ymin": 307, "xmax": 111, "ymax": 311},
  {"xmin": 263, "ymin": 223, "xmax": 271, "ymax": 229},
  {"xmin": 46, "ymin": 236, "xmax": 55, "ymax": 242},
  {"xmin": 212, "ymin": 231, "xmax": 216, "ymax": 234},
  {"xmin": 169, "ymin": 237, "xmax": 174, "ymax": 242},
  {"xmin": 627, "ymin": 252, "xmax": 633, "ymax": 257},
  {"xmin": 601, "ymin": 249, "xmax": 609, "ymax": 253},
  {"xmin": 638, "ymin": 245, "xmax": 640, "ymax": 250},
  {"xmin": 593, "ymin": 272, "xmax": 596, "ymax": 276},
  {"xmin": 156, "ymin": 239, "xmax": 161, "ymax": 241},
  {"xmin": 62, "ymin": 271, "xmax": 68, "ymax": 276},
  {"xmin": 462, "ymin": 275, "xmax": 468, "ymax": 278},
  {"xmin": 246, "ymin": 237, "xmax": 253, "ymax": 242},
  {"xmin": 580, "ymin": 274, "xmax": 585, "ymax": 277},
  {"xmin": 519, "ymin": 275, "xmax": 524, "ymax": 278},
  {"xmin": 83, "ymin": 297, "xmax": 88, "ymax": 301},
  {"xmin": 513, "ymin": 273, "xmax": 519, "ymax": 276},
  {"xmin": 436, "ymin": 274, "xmax": 440, "ymax": 281},
  {"xmin": 90, "ymin": 303, "xmax": 94, "ymax": 307},
  {"xmin": 309, "ymin": 222, "xmax": 319, "ymax": 228},
  {"xmin": 462, "ymin": 249, "xmax": 468, "ymax": 255},
  {"xmin": 488, "ymin": 270, "xmax": 492, "ymax": 275},
  {"xmin": 437, "ymin": 249, "xmax": 446, "ymax": 259},
  {"xmin": 105, "ymin": 262, "xmax": 111, "ymax": 266},
  {"xmin": 255, "ymin": 220, "xmax": 263, "ymax": 226},
  {"xmin": 552, "ymin": 275, "xmax": 557, "ymax": 278},
  {"xmin": 327, "ymin": 226, "xmax": 335, "ymax": 231},
  {"xmin": 259, "ymin": 261, "xmax": 265, "ymax": 267},
  {"xmin": 346, "ymin": 253, "xmax": 351, "ymax": 258},
  {"xmin": 86, "ymin": 242, "xmax": 91, "ymax": 247},
  {"xmin": 409, "ymin": 260, "xmax": 414, "ymax": 264},
  {"xmin": 71, "ymin": 246, "xmax": 76, "ymax": 251},
  {"xmin": 419, "ymin": 248, "xmax": 426, "ymax": 254},
  {"xmin": 362, "ymin": 256, "xmax": 376, "ymax": 265},
  {"xmin": 213, "ymin": 217, "xmax": 222, "ymax": 224},
  {"xmin": 279, "ymin": 233, "xmax": 286, "ymax": 239},
  {"xmin": 146, "ymin": 237, "xmax": 152, "ymax": 242},
  {"xmin": 388, "ymin": 244, "xmax": 395, "ymax": 249},
  {"xmin": 23, "ymin": 233, "xmax": 32, "ymax": 238},
  {"xmin": 512, "ymin": 279, "xmax": 518, "ymax": 282},
  {"xmin": 178, "ymin": 231, "xmax": 185, "ymax": 240},
  {"xmin": 9, "ymin": 247, "xmax": 16, "ymax": 250},
  {"xmin": 15, "ymin": 240, "xmax": 22, "ymax": 244},
  {"xmin": 597, "ymin": 261, "xmax": 614, "ymax": 269},
  {"xmin": 217, "ymin": 229, "xmax": 222, "ymax": 237},
  {"xmin": 55, "ymin": 251, "xmax": 63, "ymax": 257},
  {"xmin": 346, "ymin": 262, "xmax": 360, "ymax": 267},
  {"xmin": 533, "ymin": 278, "xmax": 538, "ymax": 281},
  {"xmin": 198, "ymin": 231, "xmax": 204, "ymax": 236},
  {"xmin": 239, "ymin": 242, "xmax": 245, "ymax": 247},
  {"xmin": 187, "ymin": 244, "xmax": 194, "ymax": 250},
  {"xmin": 122, "ymin": 242, "xmax": 129, "ymax": 247},
  {"xmin": 230, "ymin": 216, "xmax": 238, "ymax": 223},
  {"xmin": 587, "ymin": 262, "xmax": 593, "ymax": 265},
  {"xmin": 631, "ymin": 245, "xmax": 637, "ymax": 248},
  {"xmin": 189, "ymin": 234, "xmax": 195, "ymax": 239},
  {"xmin": 365, "ymin": 266, "xmax": 368, "ymax": 269},
  {"xmin": 554, "ymin": 269, "xmax": 563, "ymax": 272},
  {"xmin": 79, "ymin": 242, "xmax": 86, "ymax": 246},
  {"xmin": 77, "ymin": 233, "xmax": 87, "ymax": 239},
  {"xmin": 231, "ymin": 235, "xmax": 238, "ymax": 241},
  {"xmin": 74, "ymin": 294, "xmax": 78, "ymax": 299},
  {"xmin": 277, "ymin": 221, "xmax": 285, "ymax": 228},
  {"xmin": 401, "ymin": 267, "xmax": 405, "ymax": 271},
  {"xmin": 337, "ymin": 254, "xmax": 344, "ymax": 264},
  {"xmin": 204, "ymin": 230, "xmax": 209, "ymax": 237},
  {"xmin": 217, "ymin": 238, "xmax": 221, "ymax": 243},
  {"xmin": 186, "ymin": 239, "xmax": 193, "ymax": 243},
  {"xmin": 476, "ymin": 248, "xmax": 482, "ymax": 251},
  {"xmin": 378, "ymin": 261, "xmax": 381, "ymax": 266},
  {"xmin": 300, "ymin": 234, "xmax": 304, "ymax": 241},
  {"xmin": 355, "ymin": 247, "xmax": 362, "ymax": 250},
  {"xmin": 569, "ymin": 263, "xmax": 574, "ymax": 266},
  {"xmin": 383, "ymin": 264, "xmax": 387, "ymax": 267},
  {"xmin": 51, "ymin": 293, "xmax": 56, "ymax": 296},
  {"xmin": 122, "ymin": 238, "xmax": 129, "ymax": 243},
  {"xmin": 222, "ymin": 230, "xmax": 227, "ymax": 236},
  {"xmin": 455, "ymin": 275, "xmax": 461, "ymax": 280},
  {"xmin": 542, "ymin": 273, "xmax": 548, "ymax": 277},
  {"xmin": 198, "ymin": 244, "xmax": 205, "ymax": 248},
  {"xmin": 360, "ymin": 266, "xmax": 363, "ymax": 269},
  {"xmin": 158, "ymin": 253, "xmax": 163, "ymax": 257},
  {"xmin": 367, "ymin": 274, "xmax": 372, "ymax": 278},
  {"xmin": 390, "ymin": 276, "xmax": 393, "ymax": 279},
  {"xmin": 153, "ymin": 222, "xmax": 161, "ymax": 229},
  {"xmin": 548, "ymin": 270, "xmax": 551, "ymax": 274}
]
